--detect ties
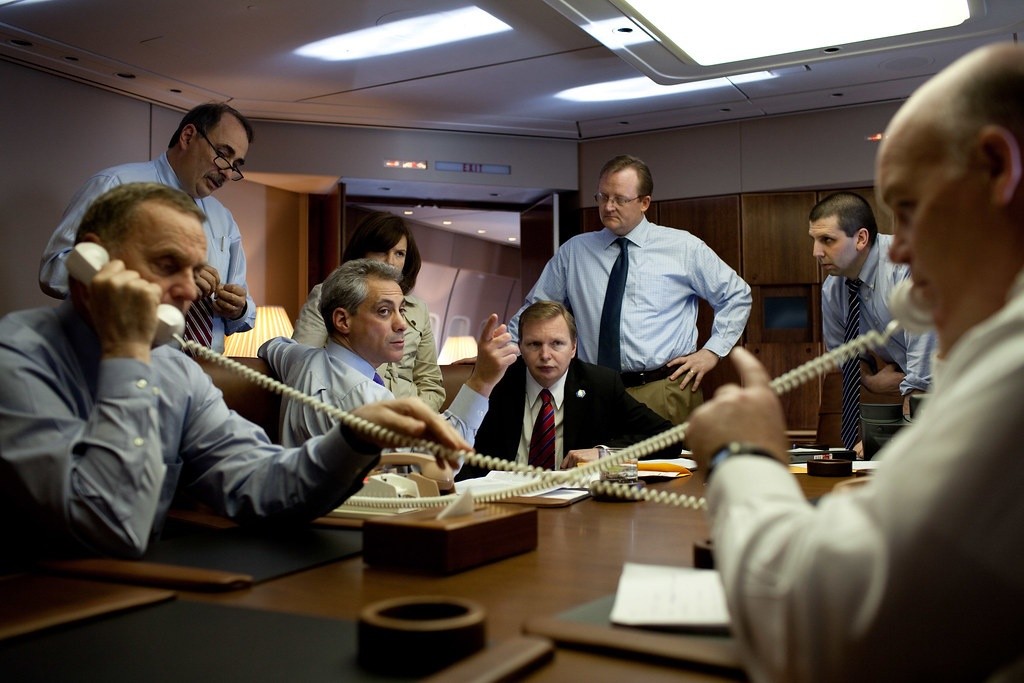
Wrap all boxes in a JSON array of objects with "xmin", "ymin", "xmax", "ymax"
[
  {"xmin": 373, "ymin": 372, "xmax": 385, "ymax": 387},
  {"xmin": 597, "ymin": 237, "xmax": 631, "ymax": 373},
  {"xmin": 185, "ymin": 193, "xmax": 214, "ymax": 357},
  {"xmin": 841, "ymin": 278, "xmax": 864, "ymax": 449},
  {"xmin": 528, "ymin": 388, "xmax": 555, "ymax": 471}
]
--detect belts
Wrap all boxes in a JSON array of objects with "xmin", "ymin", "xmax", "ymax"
[{"xmin": 621, "ymin": 354, "xmax": 691, "ymax": 389}]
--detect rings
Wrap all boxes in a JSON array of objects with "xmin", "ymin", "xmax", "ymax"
[
  {"xmin": 690, "ymin": 370, "xmax": 695, "ymax": 375},
  {"xmin": 232, "ymin": 305, "xmax": 235, "ymax": 312}
]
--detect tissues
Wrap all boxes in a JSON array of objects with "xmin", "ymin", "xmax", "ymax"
[{"xmin": 362, "ymin": 486, "xmax": 538, "ymax": 577}]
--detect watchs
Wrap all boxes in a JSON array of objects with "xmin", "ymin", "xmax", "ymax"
[{"xmin": 702, "ymin": 442, "xmax": 787, "ymax": 485}]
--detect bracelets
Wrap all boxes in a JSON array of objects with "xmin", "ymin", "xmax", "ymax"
[{"xmin": 598, "ymin": 447, "xmax": 606, "ymax": 460}]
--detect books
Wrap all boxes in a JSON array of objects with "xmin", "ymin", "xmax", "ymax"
[{"xmin": 449, "ymin": 470, "xmax": 590, "ymax": 507}]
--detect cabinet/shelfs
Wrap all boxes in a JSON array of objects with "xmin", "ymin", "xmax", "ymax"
[{"xmin": 578, "ymin": 185, "xmax": 903, "ymax": 443}]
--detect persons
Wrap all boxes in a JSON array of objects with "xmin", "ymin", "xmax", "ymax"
[
  {"xmin": 290, "ymin": 212, "xmax": 446, "ymax": 414},
  {"xmin": 257, "ymin": 259, "xmax": 521, "ymax": 481},
  {"xmin": 38, "ymin": 100, "xmax": 256, "ymax": 392},
  {"xmin": 507, "ymin": 154, "xmax": 753, "ymax": 427},
  {"xmin": 809, "ymin": 191, "xmax": 939, "ymax": 450},
  {"xmin": 461, "ymin": 300, "xmax": 684, "ymax": 481},
  {"xmin": 687, "ymin": 42, "xmax": 1024, "ymax": 683},
  {"xmin": 0, "ymin": 181, "xmax": 476, "ymax": 567}
]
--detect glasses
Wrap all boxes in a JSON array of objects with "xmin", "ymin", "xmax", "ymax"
[
  {"xmin": 195, "ymin": 127, "xmax": 244, "ymax": 181},
  {"xmin": 594, "ymin": 190, "xmax": 651, "ymax": 207}
]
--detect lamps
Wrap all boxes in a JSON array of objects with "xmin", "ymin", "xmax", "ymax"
[{"xmin": 546, "ymin": 0, "xmax": 987, "ymax": 86}]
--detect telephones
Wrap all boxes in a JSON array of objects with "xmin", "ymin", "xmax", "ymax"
[
  {"xmin": 375, "ymin": 453, "xmax": 456, "ymax": 497},
  {"xmin": 889, "ymin": 279, "xmax": 936, "ymax": 335},
  {"xmin": 65, "ymin": 242, "xmax": 186, "ymax": 346}
]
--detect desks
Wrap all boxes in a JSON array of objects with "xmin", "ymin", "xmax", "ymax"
[{"xmin": 1, "ymin": 457, "xmax": 875, "ymax": 683}]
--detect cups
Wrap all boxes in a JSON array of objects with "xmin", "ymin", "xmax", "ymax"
[{"xmin": 598, "ymin": 449, "xmax": 638, "ymax": 483}]
